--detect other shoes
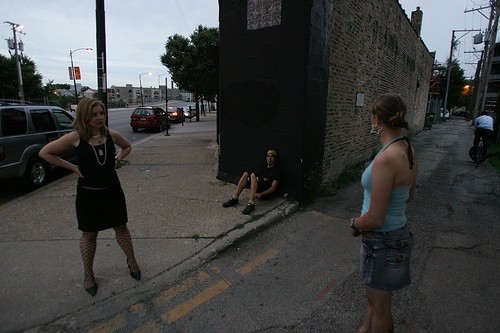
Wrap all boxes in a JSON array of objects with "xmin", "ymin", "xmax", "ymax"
[
  {"xmin": 222, "ymin": 198, "xmax": 239, "ymax": 208},
  {"xmin": 242, "ymin": 202, "xmax": 255, "ymax": 215}
]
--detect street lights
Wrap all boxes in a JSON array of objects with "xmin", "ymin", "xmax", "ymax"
[
  {"xmin": 69, "ymin": 47, "xmax": 92, "ymax": 104},
  {"xmin": 139, "ymin": 72, "xmax": 151, "ymax": 106}
]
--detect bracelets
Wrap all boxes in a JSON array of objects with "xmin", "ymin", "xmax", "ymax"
[{"xmin": 350, "ymin": 218, "xmax": 354, "ymax": 227}]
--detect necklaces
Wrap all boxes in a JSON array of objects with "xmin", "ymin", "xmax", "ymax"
[{"xmin": 89, "ymin": 137, "xmax": 107, "ymax": 165}]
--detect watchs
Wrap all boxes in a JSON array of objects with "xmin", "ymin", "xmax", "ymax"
[{"xmin": 115, "ymin": 156, "xmax": 120, "ymax": 162}]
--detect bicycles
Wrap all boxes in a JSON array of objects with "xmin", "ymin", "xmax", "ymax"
[{"xmin": 469, "ymin": 138, "xmax": 483, "ymax": 169}]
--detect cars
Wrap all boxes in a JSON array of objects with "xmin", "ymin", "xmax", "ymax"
[
  {"xmin": 430, "ymin": 108, "xmax": 449, "ymax": 121},
  {"xmin": 164, "ymin": 106, "xmax": 196, "ymax": 123},
  {"xmin": 130, "ymin": 106, "xmax": 170, "ymax": 133}
]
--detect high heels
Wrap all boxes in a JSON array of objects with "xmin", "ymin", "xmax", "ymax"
[
  {"xmin": 127, "ymin": 258, "xmax": 141, "ymax": 280},
  {"xmin": 84, "ymin": 272, "xmax": 97, "ymax": 295}
]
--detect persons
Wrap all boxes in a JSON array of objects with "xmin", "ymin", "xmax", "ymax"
[
  {"xmin": 350, "ymin": 93, "xmax": 416, "ymax": 333},
  {"xmin": 40, "ymin": 98, "xmax": 141, "ymax": 297},
  {"xmin": 222, "ymin": 147, "xmax": 281, "ymax": 215},
  {"xmin": 472, "ymin": 111, "xmax": 494, "ymax": 159}
]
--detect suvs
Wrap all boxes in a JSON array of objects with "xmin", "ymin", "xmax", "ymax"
[{"xmin": 0, "ymin": 98, "xmax": 75, "ymax": 192}]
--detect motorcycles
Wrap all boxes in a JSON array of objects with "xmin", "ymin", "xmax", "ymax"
[{"xmin": 424, "ymin": 112, "xmax": 436, "ymax": 130}]
